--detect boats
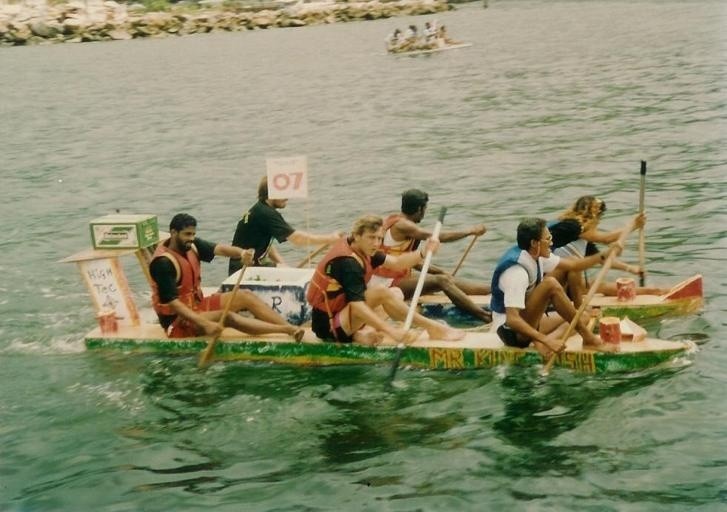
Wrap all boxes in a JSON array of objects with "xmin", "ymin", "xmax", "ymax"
[
  {"xmin": 385, "ymin": 43, "xmax": 471, "ymax": 56},
  {"xmin": 55, "ymin": 231, "xmax": 705, "ymax": 376}
]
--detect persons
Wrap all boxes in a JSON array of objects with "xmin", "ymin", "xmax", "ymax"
[
  {"xmin": 229, "ymin": 173, "xmax": 346, "ymax": 278},
  {"xmin": 308, "ymin": 189, "xmax": 493, "ymax": 346},
  {"xmin": 490, "ymin": 193, "xmax": 648, "ymax": 359},
  {"xmin": 149, "ymin": 212, "xmax": 304, "ymax": 343},
  {"xmin": 387, "ymin": 22, "xmax": 462, "ymax": 52}
]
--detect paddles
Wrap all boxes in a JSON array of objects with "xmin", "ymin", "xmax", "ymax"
[{"xmin": 387, "ymin": 207, "xmax": 447, "ymax": 379}]
